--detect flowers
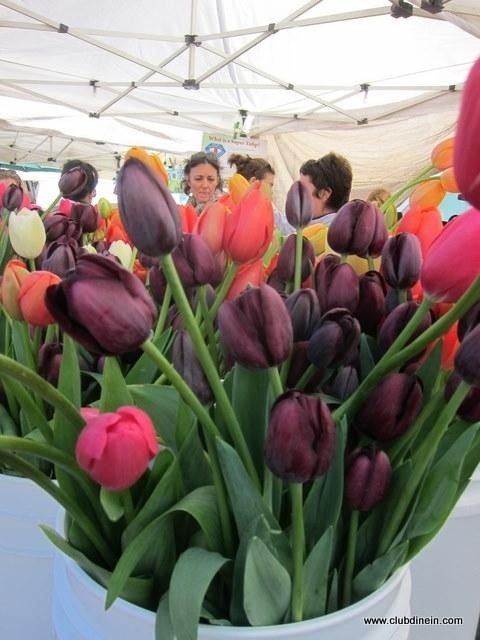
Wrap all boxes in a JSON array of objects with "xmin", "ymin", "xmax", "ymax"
[
  {"xmin": 0, "ymin": 160, "xmax": 122, "ymax": 479},
  {"xmin": 0, "ymin": 59, "xmax": 480, "ymax": 640}
]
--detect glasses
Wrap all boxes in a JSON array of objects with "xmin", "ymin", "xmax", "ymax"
[{"xmin": 192, "ymin": 151, "xmax": 216, "ymax": 162}]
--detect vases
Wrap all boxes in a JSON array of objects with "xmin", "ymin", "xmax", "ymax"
[
  {"xmin": 48, "ymin": 485, "xmax": 414, "ymax": 640},
  {"xmin": 1, "ymin": 474, "xmax": 66, "ymax": 640}
]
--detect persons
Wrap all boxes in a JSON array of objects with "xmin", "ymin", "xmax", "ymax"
[
  {"xmin": 272, "ymin": 152, "xmax": 355, "ymax": 239},
  {"xmin": 180, "ymin": 148, "xmax": 227, "ymax": 209},
  {"xmin": 226, "ymin": 152, "xmax": 275, "ymax": 207},
  {"xmin": 367, "ymin": 187, "xmax": 403, "ymax": 222},
  {"xmin": 54, "ymin": 158, "xmax": 102, "ymax": 208},
  {"xmin": 0, "ymin": 168, "xmax": 45, "ymax": 219}
]
